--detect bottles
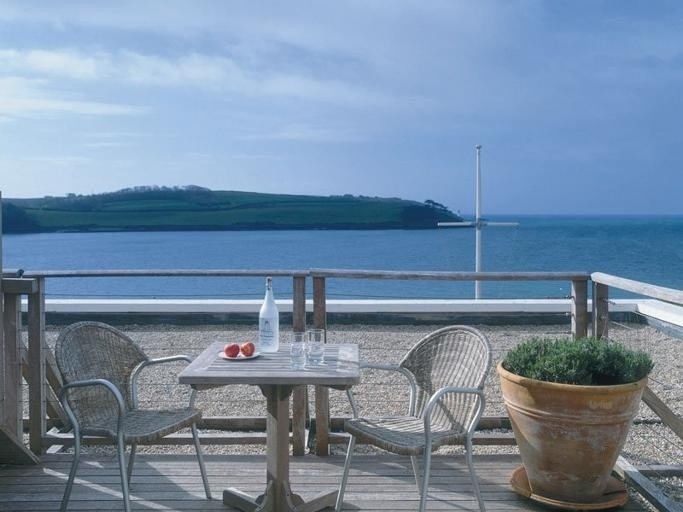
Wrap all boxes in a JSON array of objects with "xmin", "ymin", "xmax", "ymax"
[{"xmin": 259, "ymin": 277, "xmax": 279, "ymax": 353}]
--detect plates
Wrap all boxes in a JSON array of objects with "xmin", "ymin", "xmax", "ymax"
[{"xmin": 218, "ymin": 351, "xmax": 258, "ymax": 360}]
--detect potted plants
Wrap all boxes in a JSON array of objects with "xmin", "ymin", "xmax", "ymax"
[{"xmin": 497, "ymin": 334, "xmax": 654, "ymax": 502}]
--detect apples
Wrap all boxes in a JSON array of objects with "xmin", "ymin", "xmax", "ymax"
[
  {"xmin": 224, "ymin": 343, "xmax": 239, "ymax": 357},
  {"xmin": 241, "ymin": 342, "xmax": 254, "ymax": 356}
]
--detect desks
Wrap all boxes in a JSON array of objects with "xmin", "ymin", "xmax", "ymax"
[{"xmin": 178, "ymin": 340, "xmax": 360, "ymax": 512}]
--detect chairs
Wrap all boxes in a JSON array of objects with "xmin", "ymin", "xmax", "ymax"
[
  {"xmin": 56, "ymin": 321, "xmax": 212, "ymax": 512},
  {"xmin": 335, "ymin": 324, "xmax": 492, "ymax": 512}
]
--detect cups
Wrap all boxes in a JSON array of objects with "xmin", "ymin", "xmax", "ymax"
[{"xmin": 290, "ymin": 328, "xmax": 325, "ymax": 371}]
